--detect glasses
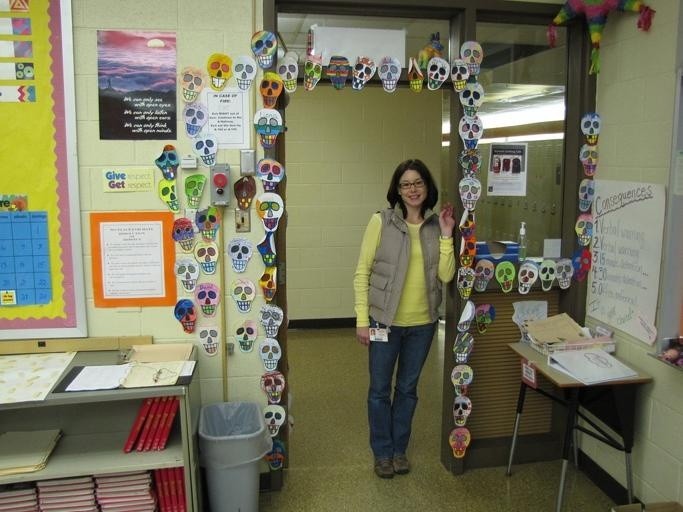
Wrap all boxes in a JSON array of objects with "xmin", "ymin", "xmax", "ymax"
[{"xmin": 397, "ymin": 180, "xmax": 425, "ymax": 189}]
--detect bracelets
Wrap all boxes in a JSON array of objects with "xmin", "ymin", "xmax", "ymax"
[{"xmin": 440, "ymin": 236, "xmax": 450, "ymax": 239}]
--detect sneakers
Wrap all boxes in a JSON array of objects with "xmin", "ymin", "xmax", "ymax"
[
  {"xmin": 373, "ymin": 458, "xmax": 394, "ymax": 479},
  {"xmin": 392, "ymin": 454, "xmax": 409, "ymax": 474}
]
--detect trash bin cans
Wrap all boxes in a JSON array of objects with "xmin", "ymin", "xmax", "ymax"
[{"xmin": 198, "ymin": 401, "xmax": 265, "ymax": 512}]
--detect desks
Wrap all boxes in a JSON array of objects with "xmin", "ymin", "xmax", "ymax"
[{"xmin": 504, "ymin": 341, "xmax": 656, "ymax": 511}]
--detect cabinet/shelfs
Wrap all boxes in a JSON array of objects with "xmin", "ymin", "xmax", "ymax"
[
  {"xmin": 1, "ymin": 343, "xmax": 203, "ymax": 512},
  {"xmin": 474, "ymin": 138, "xmax": 564, "ymax": 261}
]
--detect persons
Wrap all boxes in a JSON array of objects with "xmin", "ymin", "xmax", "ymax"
[
  {"xmin": 351, "ymin": 158, "xmax": 455, "ymax": 477},
  {"xmin": 371, "ymin": 329, "xmax": 375, "ymax": 335}
]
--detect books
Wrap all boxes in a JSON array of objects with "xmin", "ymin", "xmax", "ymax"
[
  {"xmin": 0, "ymin": 396, "xmax": 187, "ymax": 512},
  {"xmin": 521, "ymin": 311, "xmax": 590, "ymax": 349},
  {"xmin": 50, "ymin": 359, "xmax": 198, "ymax": 394}
]
